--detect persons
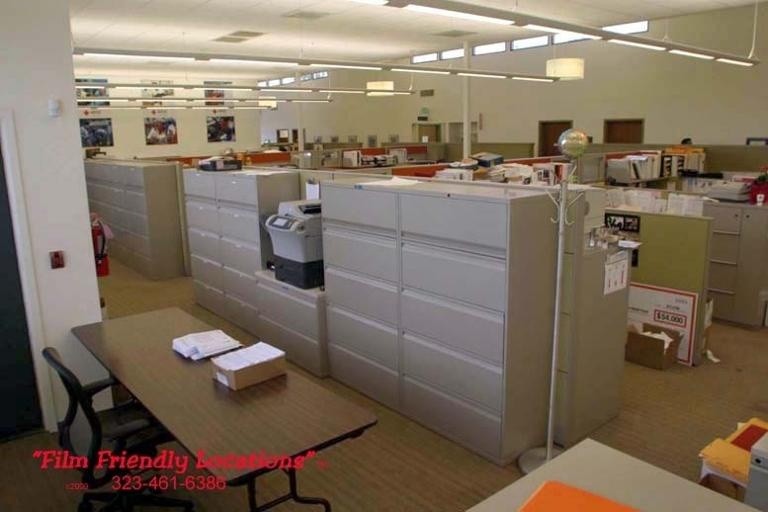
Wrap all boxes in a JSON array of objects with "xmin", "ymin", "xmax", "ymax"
[
  {"xmin": 208, "ymin": 117, "xmax": 232, "ymax": 141},
  {"xmin": 145, "ymin": 118, "xmax": 175, "ymax": 144}
]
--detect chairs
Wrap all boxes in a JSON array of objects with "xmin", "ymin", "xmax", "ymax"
[{"xmin": 40, "ymin": 343, "xmax": 198, "ymax": 510}]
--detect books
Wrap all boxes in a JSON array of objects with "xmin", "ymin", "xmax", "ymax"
[
  {"xmin": 606, "ymin": 148, "xmax": 706, "ymax": 182},
  {"xmin": 343, "ymin": 149, "xmax": 407, "ymax": 168},
  {"xmin": 435, "ymin": 162, "xmax": 573, "ymax": 186}
]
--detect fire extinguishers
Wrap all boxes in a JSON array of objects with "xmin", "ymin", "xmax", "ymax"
[{"xmin": 91, "ymin": 214, "xmax": 110, "ymax": 276}]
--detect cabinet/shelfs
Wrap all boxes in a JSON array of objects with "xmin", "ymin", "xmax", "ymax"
[
  {"xmin": 85, "ymin": 154, "xmax": 182, "ymax": 283},
  {"xmin": 710, "ymin": 201, "xmax": 767, "ymax": 330},
  {"xmin": 181, "ymin": 166, "xmax": 298, "ymax": 338},
  {"xmin": 254, "ymin": 268, "xmax": 327, "ymax": 379},
  {"xmin": 322, "ymin": 175, "xmax": 587, "ymax": 469}
]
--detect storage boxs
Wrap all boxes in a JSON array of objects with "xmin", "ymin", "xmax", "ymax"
[
  {"xmin": 212, "ymin": 339, "xmax": 289, "ymax": 392},
  {"xmin": 627, "ymin": 318, "xmax": 684, "ymax": 369}
]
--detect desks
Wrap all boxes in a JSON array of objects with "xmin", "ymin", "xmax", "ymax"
[
  {"xmin": 464, "ymin": 435, "xmax": 757, "ymax": 512},
  {"xmin": 71, "ymin": 305, "xmax": 380, "ymax": 512}
]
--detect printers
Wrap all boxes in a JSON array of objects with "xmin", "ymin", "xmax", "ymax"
[{"xmin": 265, "ymin": 199, "xmax": 324, "ymax": 290}]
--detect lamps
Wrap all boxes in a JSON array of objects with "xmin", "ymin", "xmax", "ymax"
[
  {"xmin": 366, "ymin": 81, "xmax": 395, "ymax": 96},
  {"xmin": 258, "ymin": 94, "xmax": 279, "ymax": 107},
  {"xmin": 545, "ymin": 57, "xmax": 585, "ymax": 81}
]
